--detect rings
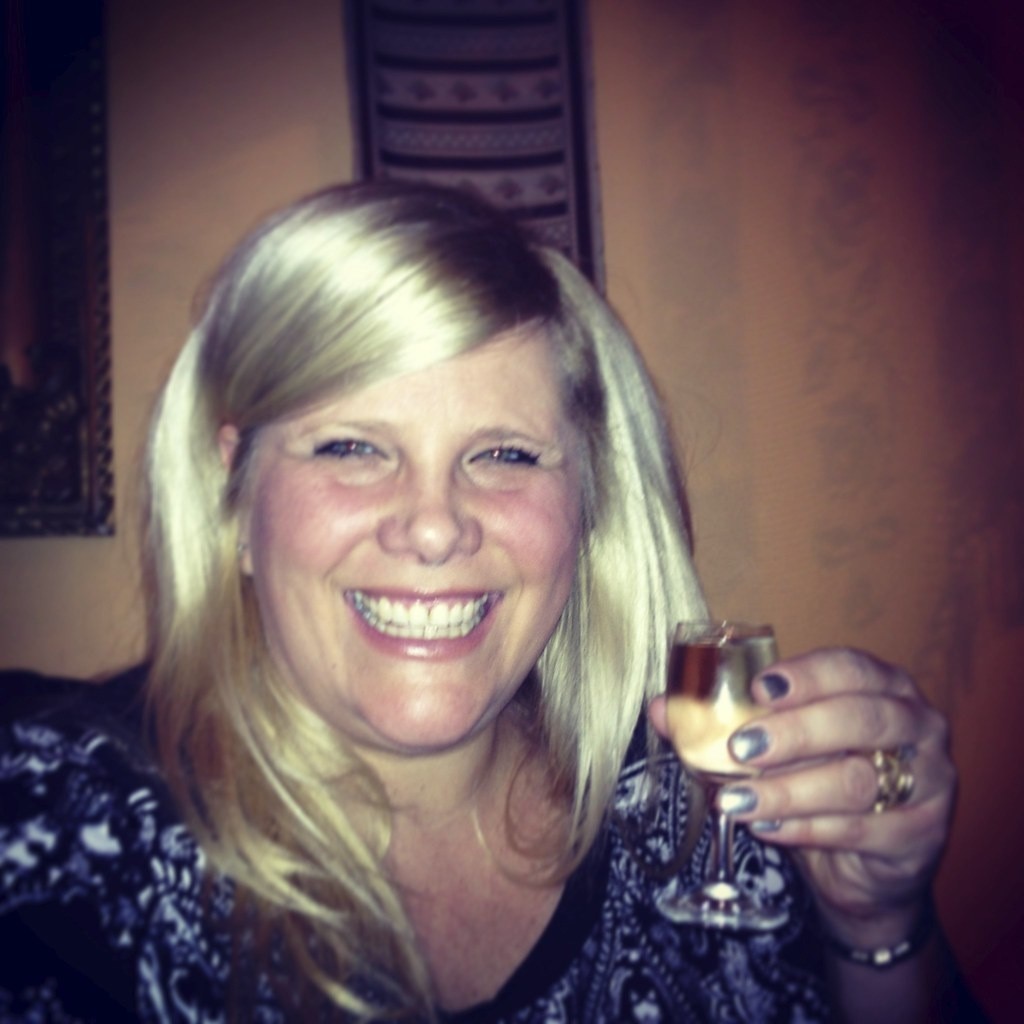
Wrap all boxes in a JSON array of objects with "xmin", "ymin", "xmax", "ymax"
[{"xmin": 870, "ymin": 750, "xmax": 918, "ymax": 815}]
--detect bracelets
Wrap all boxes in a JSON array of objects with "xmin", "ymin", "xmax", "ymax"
[{"xmin": 826, "ymin": 902, "xmax": 929, "ymax": 969}]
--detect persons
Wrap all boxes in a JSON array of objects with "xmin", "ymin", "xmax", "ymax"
[{"xmin": 0, "ymin": 177, "xmax": 977, "ymax": 1024}]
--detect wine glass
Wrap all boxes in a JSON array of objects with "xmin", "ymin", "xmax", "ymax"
[{"xmin": 655, "ymin": 621, "xmax": 791, "ymax": 931}]
